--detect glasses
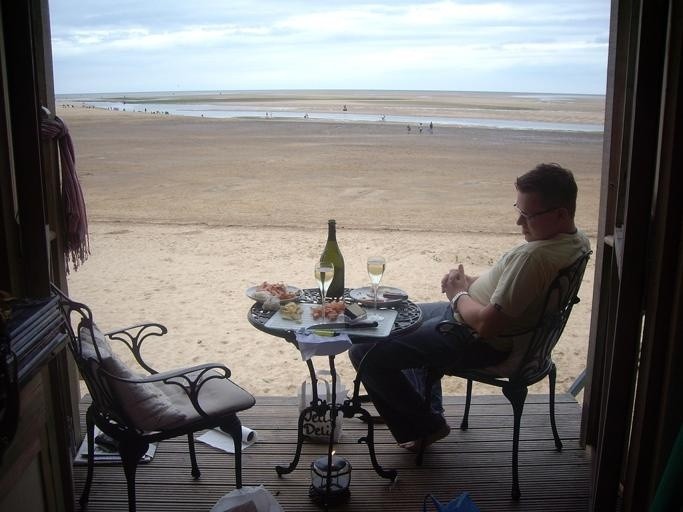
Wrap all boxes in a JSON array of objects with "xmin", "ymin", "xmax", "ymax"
[{"xmin": 513, "ymin": 203, "xmax": 569, "ymax": 220}]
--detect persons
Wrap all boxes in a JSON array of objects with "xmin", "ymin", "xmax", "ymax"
[
  {"xmin": 348, "ymin": 161, "xmax": 591, "ymax": 454},
  {"xmin": 418, "ymin": 123, "xmax": 423, "ymax": 133},
  {"xmin": 430, "ymin": 122, "xmax": 433, "ymax": 134},
  {"xmin": 407, "ymin": 126, "xmax": 411, "ymax": 134}
]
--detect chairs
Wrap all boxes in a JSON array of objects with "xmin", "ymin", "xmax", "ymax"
[
  {"xmin": 416, "ymin": 249, "xmax": 593, "ymax": 499},
  {"xmin": 50, "ymin": 282, "xmax": 256, "ymax": 512}
]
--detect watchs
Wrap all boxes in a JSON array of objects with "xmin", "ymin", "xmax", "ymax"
[{"xmin": 450, "ymin": 291, "xmax": 470, "ymax": 313}]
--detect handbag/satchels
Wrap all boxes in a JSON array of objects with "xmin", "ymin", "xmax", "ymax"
[
  {"xmin": 298, "ymin": 369, "xmax": 349, "ymax": 443},
  {"xmin": 424, "ymin": 492, "xmax": 479, "ymax": 512}
]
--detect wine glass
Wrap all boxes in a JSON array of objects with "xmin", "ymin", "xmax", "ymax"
[
  {"xmin": 367, "ymin": 257, "xmax": 387, "ymax": 323},
  {"xmin": 313, "ymin": 261, "xmax": 335, "ymax": 332}
]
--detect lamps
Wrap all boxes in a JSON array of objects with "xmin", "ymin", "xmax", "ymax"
[{"xmin": 309, "ymin": 449, "xmax": 352, "ymax": 505}]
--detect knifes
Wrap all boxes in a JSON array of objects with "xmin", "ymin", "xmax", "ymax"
[{"xmin": 307, "ymin": 322, "xmax": 377, "ymax": 330}]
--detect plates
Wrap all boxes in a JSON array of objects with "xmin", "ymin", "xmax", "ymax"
[
  {"xmin": 350, "ymin": 285, "xmax": 408, "ymax": 308},
  {"xmin": 244, "ymin": 283, "xmax": 304, "ymax": 305}
]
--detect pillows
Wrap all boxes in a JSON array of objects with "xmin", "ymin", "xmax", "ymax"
[
  {"xmin": 497, "ymin": 245, "xmax": 588, "ymax": 378},
  {"xmin": 76, "ymin": 318, "xmax": 186, "ymax": 431}
]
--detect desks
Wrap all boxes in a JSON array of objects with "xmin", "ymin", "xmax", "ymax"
[{"xmin": 247, "ymin": 287, "xmax": 424, "ymax": 512}]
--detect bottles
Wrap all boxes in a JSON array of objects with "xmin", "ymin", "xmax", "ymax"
[{"xmin": 317, "ymin": 218, "xmax": 344, "ymax": 302}]
